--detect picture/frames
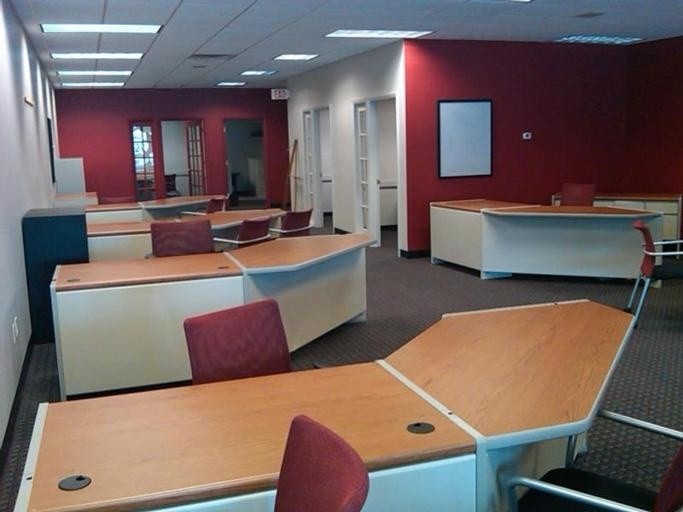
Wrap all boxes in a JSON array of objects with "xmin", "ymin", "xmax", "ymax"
[{"xmin": 435, "ymin": 97, "xmax": 494, "ymax": 179}]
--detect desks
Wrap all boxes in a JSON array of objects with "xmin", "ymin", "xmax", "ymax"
[
  {"xmin": 8, "ymin": 292, "xmax": 638, "ymax": 512},
  {"xmin": 426, "ymin": 196, "xmax": 665, "ymax": 292}
]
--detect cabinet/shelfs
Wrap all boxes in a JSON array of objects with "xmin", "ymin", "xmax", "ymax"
[{"xmin": 550, "ymin": 190, "xmax": 683, "ymax": 261}]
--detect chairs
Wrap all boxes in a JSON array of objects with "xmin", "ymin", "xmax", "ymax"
[
  {"xmin": 501, "ymin": 405, "xmax": 683, "ymax": 512},
  {"xmin": 40, "ymin": 171, "xmax": 378, "ymax": 403},
  {"xmin": 558, "ymin": 181, "xmax": 597, "ymax": 208},
  {"xmin": 269, "ymin": 413, "xmax": 372, "ymax": 512},
  {"xmin": 621, "ymin": 220, "xmax": 683, "ymax": 329}
]
